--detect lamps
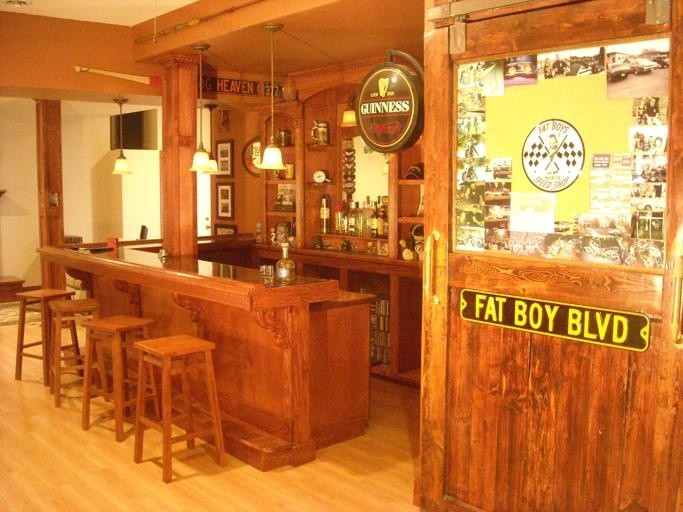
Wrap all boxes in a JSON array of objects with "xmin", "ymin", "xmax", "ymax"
[
  {"xmin": 112, "ymin": 98, "xmax": 131, "ymax": 175},
  {"xmin": 49, "ymin": 299, "xmax": 109, "ymax": 407},
  {"xmin": 82, "ymin": 316, "xmax": 160, "ymax": 442},
  {"xmin": 260, "ymin": 24, "xmax": 286, "ymax": 170},
  {"xmin": 189, "ymin": 44, "xmax": 220, "ymax": 175},
  {"xmin": 133, "ymin": 334, "xmax": 228, "ymax": 483},
  {"xmin": 15, "ymin": 288, "xmax": 84, "ymax": 386}
]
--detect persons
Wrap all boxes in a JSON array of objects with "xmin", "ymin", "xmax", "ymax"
[{"xmin": 454, "ymin": 49, "xmax": 666, "ymax": 265}]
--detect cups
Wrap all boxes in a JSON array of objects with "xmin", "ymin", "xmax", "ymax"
[
  {"xmin": 286, "ymin": 164, "xmax": 293, "ymax": 179},
  {"xmin": 160, "ymin": 249, "xmax": 168, "ymax": 256},
  {"xmin": 311, "ymin": 122, "xmax": 329, "ymax": 146},
  {"xmin": 259, "ymin": 265, "xmax": 273, "ymax": 287}
]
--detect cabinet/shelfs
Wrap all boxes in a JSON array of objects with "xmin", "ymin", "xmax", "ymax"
[
  {"xmin": 251, "ymin": 244, "xmax": 424, "ymax": 390},
  {"xmin": 264, "ymin": 78, "xmax": 424, "ymax": 263}
]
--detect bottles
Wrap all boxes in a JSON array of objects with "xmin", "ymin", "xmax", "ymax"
[
  {"xmin": 312, "ymin": 238, "xmax": 388, "ymax": 256},
  {"xmin": 319, "ymin": 194, "xmax": 389, "ymax": 238},
  {"xmin": 274, "ymin": 195, "xmax": 282, "ymax": 212},
  {"xmin": 282, "ymin": 189, "xmax": 293, "ymax": 212},
  {"xmin": 256, "ymin": 217, "xmax": 295, "ymax": 247},
  {"xmin": 276, "ymin": 242, "xmax": 295, "ymax": 282},
  {"xmin": 50, "ymin": 192, "xmax": 58, "ymax": 208},
  {"xmin": 279, "ymin": 129, "xmax": 288, "ymax": 147}
]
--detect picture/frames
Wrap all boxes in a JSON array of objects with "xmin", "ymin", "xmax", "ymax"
[
  {"xmin": 213, "ymin": 223, "xmax": 237, "ymax": 235},
  {"xmin": 215, "ymin": 183, "xmax": 234, "ymax": 220},
  {"xmin": 215, "ymin": 139, "xmax": 234, "ymax": 178}
]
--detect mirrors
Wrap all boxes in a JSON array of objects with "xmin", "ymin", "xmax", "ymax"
[{"xmin": 242, "ymin": 136, "xmax": 263, "ymax": 177}]
